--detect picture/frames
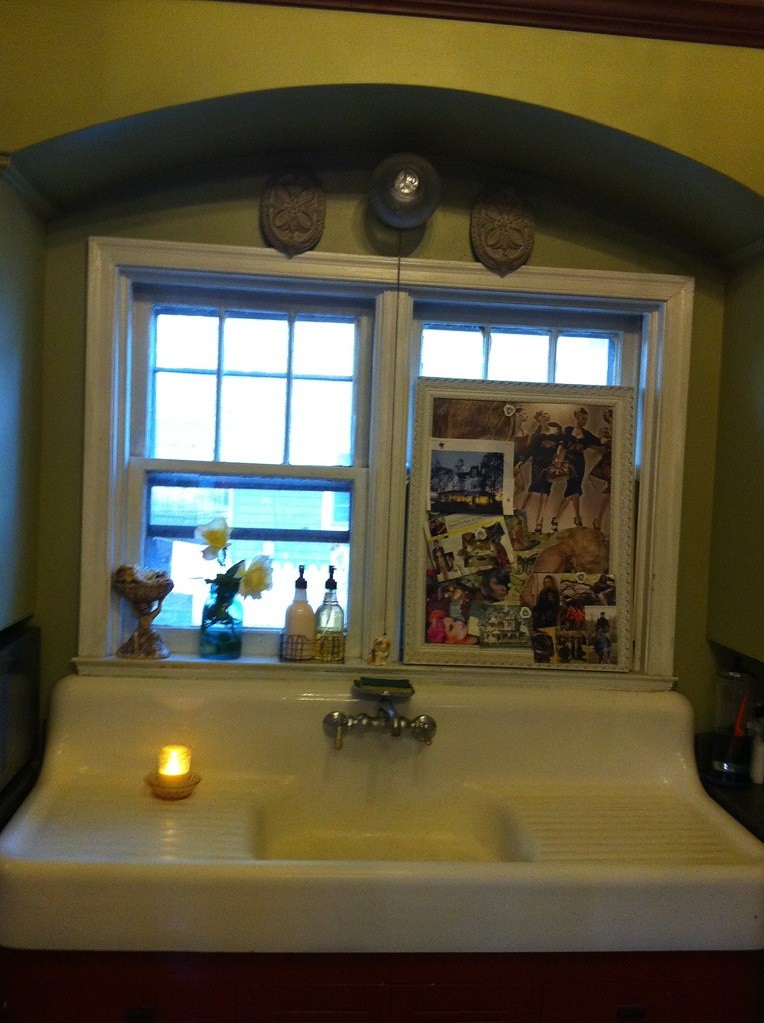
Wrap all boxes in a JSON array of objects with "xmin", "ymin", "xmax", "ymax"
[{"xmin": 403, "ymin": 377, "xmax": 635, "ymax": 675}]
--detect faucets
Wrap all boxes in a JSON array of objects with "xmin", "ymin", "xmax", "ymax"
[{"xmin": 380, "ymin": 699, "xmax": 402, "ymax": 738}]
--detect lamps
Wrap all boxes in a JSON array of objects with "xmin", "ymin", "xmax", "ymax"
[{"xmin": 369, "ymin": 149, "xmax": 441, "ymax": 226}]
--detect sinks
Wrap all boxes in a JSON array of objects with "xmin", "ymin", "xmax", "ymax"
[{"xmin": 242, "ymin": 787, "xmax": 544, "ymax": 871}]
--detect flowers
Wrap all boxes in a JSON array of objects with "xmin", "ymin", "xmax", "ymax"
[{"xmin": 194, "ymin": 516, "xmax": 274, "ymax": 630}]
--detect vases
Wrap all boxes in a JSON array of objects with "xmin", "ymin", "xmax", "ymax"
[{"xmin": 198, "ymin": 582, "xmax": 241, "ymax": 660}]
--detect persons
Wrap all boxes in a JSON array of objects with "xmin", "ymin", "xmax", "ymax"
[
  {"xmin": 432, "ymin": 540, "xmax": 451, "ymax": 579},
  {"xmin": 482, "ymin": 567, "xmax": 511, "ymax": 602},
  {"xmin": 591, "ymin": 611, "xmax": 611, "ymax": 640},
  {"xmin": 533, "ymin": 575, "xmax": 560, "ymax": 628},
  {"xmin": 514, "ymin": 407, "xmax": 613, "ymax": 534}
]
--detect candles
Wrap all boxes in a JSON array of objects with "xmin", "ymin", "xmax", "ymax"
[{"xmin": 156, "ymin": 743, "xmax": 192, "ymax": 784}]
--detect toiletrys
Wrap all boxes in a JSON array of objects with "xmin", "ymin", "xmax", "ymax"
[{"xmin": 279, "ymin": 559, "xmax": 348, "ymax": 664}]
